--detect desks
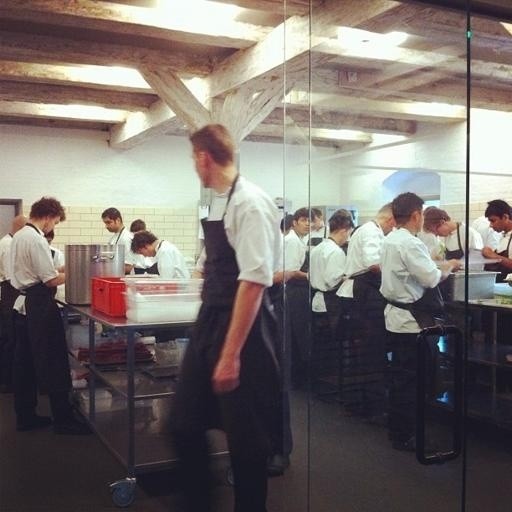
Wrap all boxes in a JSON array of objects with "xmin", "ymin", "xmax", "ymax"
[{"xmin": 450, "ymin": 300, "xmax": 512, "ymax": 441}]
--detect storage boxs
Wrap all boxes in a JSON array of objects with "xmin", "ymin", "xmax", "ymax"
[
  {"xmin": 91, "ymin": 276, "xmax": 206, "ymax": 320},
  {"xmin": 447, "ymin": 270, "xmax": 502, "ymax": 301}
]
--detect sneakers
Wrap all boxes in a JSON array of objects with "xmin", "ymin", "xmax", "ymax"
[
  {"xmin": 52, "ymin": 419, "xmax": 94, "ymax": 436},
  {"xmin": 15, "ymin": 412, "xmax": 50, "ymax": 432}
]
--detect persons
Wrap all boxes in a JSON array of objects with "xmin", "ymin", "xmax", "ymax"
[
  {"xmin": 102, "ymin": 207, "xmax": 135, "ymax": 274},
  {"xmin": 169, "ymin": 123, "xmax": 293, "ymax": 512},
  {"xmin": 129, "ymin": 219, "xmax": 159, "ymax": 275},
  {"xmin": 9, "ymin": 195, "xmax": 91, "ymax": 434},
  {"xmin": 0, "ymin": 215, "xmax": 28, "ymax": 393},
  {"xmin": 44, "ymin": 227, "xmax": 65, "ymax": 297},
  {"xmin": 131, "ymin": 230, "xmax": 190, "ymax": 279},
  {"xmin": 275, "ymin": 191, "xmax": 512, "ymax": 464}
]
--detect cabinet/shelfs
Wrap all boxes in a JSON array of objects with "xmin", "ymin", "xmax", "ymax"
[{"xmin": 54, "ymin": 299, "xmax": 239, "ymax": 508}]
